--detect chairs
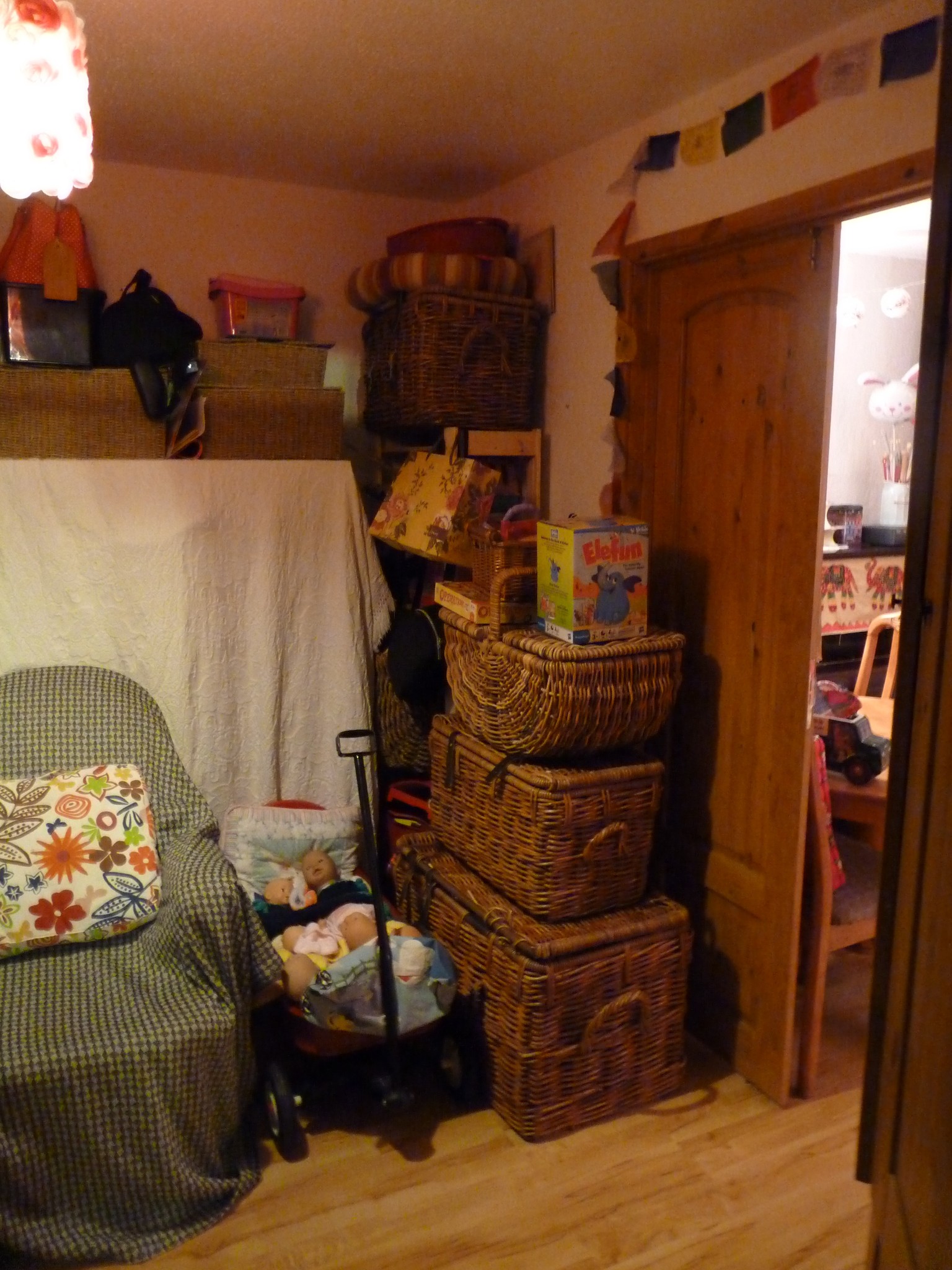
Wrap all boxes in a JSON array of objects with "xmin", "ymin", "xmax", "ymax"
[
  {"xmin": 796, "ymin": 733, "xmax": 885, "ymax": 1101},
  {"xmin": 853, "ymin": 610, "xmax": 902, "ymax": 699}
]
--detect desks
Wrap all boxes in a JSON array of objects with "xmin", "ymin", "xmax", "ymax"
[
  {"xmin": 825, "ymin": 696, "xmax": 894, "ymax": 950},
  {"xmin": 814, "ymin": 539, "xmax": 906, "ymax": 675}
]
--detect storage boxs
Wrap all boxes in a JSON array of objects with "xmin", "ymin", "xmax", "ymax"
[
  {"xmin": 435, "ymin": 581, "xmax": 538, "ymax": 624},
  {"xmin": 536, "ymin": 512, "xmax": 649, "ymax": 646},
  {"xmin": 208, "ymin": 272, "xmax": 306, "ymax": 342},
  {"xmin": 0, "ymin": 281, "xmax": 108, "ymax": 369}
]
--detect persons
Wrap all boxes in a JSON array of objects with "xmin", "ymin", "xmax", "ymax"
[{"xmin": 247, "ymin": 847, "xmax": 434, "ymax": 1014}]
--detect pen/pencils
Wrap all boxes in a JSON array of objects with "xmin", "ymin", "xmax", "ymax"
[{"xmin": 879, "ymin": 422, "xmax": 916, "ymax": 482}]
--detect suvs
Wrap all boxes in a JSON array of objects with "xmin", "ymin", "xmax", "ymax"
[{"xmin": 810, "ymin": 707, "xmax": 892, "ymax": 786}]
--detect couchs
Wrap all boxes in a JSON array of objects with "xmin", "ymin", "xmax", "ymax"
[{"xmin": 0, "ymin": 666, "xmax": 284, "ymax": 1270}]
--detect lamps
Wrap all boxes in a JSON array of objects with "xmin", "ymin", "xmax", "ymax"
[{"xmin": 0, "ymin": 0, "xmax": 95, "ymax": 201}]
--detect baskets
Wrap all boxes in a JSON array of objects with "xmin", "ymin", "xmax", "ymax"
[
  {"xmin": 426, "ymin": 707, "xmax": 666, "ymax": 921},
  {"xmin": 364, "ymin": 290, "xmax": 539, "ymax": 435},
  {"xmin": 437, "ymin": 566, "xmax": 682, "ymax": 756},
  {"xmin": 389, "ymin": 829, "xmax": 694, "ymax": 1141},
  {"xmin": 465, "ymin": 501, "xmax": 542, "ymax": 597}
]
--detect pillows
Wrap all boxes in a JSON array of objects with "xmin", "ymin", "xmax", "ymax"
[{"xmin": 0, "ymin": 763, "xmax": 162, "ymax": 962}]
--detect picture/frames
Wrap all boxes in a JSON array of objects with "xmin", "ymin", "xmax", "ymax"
[{"xmin": 508, "ymin": 226, "xmax": 556, "ymax": 318}]
